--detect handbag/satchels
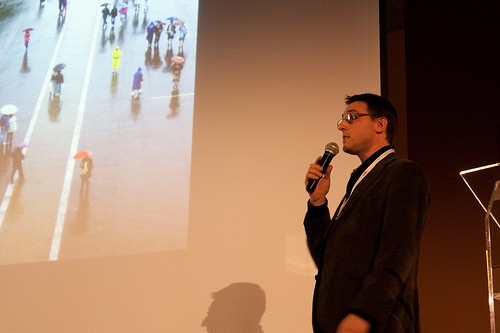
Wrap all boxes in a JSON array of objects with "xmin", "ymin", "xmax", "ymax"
[{"xmin": 81, "ymin": 161, "xmax": 89, "ymax": 177}]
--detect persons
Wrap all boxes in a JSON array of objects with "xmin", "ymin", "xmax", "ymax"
[
  {"xmin": 40, "ymin": 0, "xmax": 45, "ymax": 7},
  {"xmin": 10, "ymin": 147, "xmax": 25, "ymax": 184},
  {"xmin": 172, "ymin": 63, "xmax": 183, "ymax": 90},
  {"xmin": 6, "ymin": 114, "xmax": 17, "ymax": 145},
  {"xmin": 102, "ymin": 0, "xmax": 148, "ymax": 27},
  {"xmin": 50, "ymin": 72, "xmax": 57, "ymax": 97},
  {"xmin": 24, "ymin": 31, "xmax": 30, "ymax": 50},
  {"xmin": 0, "ymin": 115, "xmax": 10, "ymax": 145},
  {"xmin": 54, "ymin": 71, "xmax": 64, "ymax": 99},
  {"xmin": 146, "ymin": 20, "xmax": 187, "ymax": 48},
  {"xmin": 303, "ymin": 93, "xmax": 429, "ymax": 333},
  {"xmin": 111, "ymin": 45, "xmax": 121, "ymax": 73},
  {"xmin": 58, "ymin": 0, "xmax": 68, "ymax": 16},
  {"xmin": 80, "ymin": 159, "xmax": 93, "ymax": 198},
  {"xmin": 131, "ymin": 67, "xmax": 143, "ymax": 96}
]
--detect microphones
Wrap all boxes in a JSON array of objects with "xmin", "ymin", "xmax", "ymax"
[{"xmin": 305, "ymin": 142, "xmax": 339, "ymax": 193}]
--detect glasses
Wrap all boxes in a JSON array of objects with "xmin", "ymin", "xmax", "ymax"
[{"xmin": 337, "ymin": 113, "xmax": 372, "ymax": 127}]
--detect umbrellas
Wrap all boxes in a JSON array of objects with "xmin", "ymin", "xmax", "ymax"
[
  {"xmin": 73, "ymin": 151, "xmax": 93, "ymax": 159},
  {"xmin": 100, "ymin": 2, "xmax": 110, "ymax": 6},
  {"xmin": 13, "ymin": 143, "xmax": 29, "ymax": 148},
  {"xmin": 174, "ymin": 20, "xmax": 186, "ymax": 25},
  {"xmin": 53, "ymin": 63, "xmax": 66, "ymax": 72},
  {"xmin": 156, "ymin": 20, "xmax": 166, "ymax": 26},
  {"xmin": 171, "ymin": 56, "xmax": 184, "ymax": 64},
  {"xmin": 0, "ymin": 104, "xmax": 17, "ymax": 115},
  {"xmin": 22, "ymin": 28, "xmax": 34, "ymax": 32},
  {"xmin": 167, "ymin": 17, "xmax": 180, "ymax": 21}
]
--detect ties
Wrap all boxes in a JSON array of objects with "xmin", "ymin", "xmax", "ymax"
[{"xmin": 346, "ymin": 168, "xmax": 358, "ymax": 200}]
